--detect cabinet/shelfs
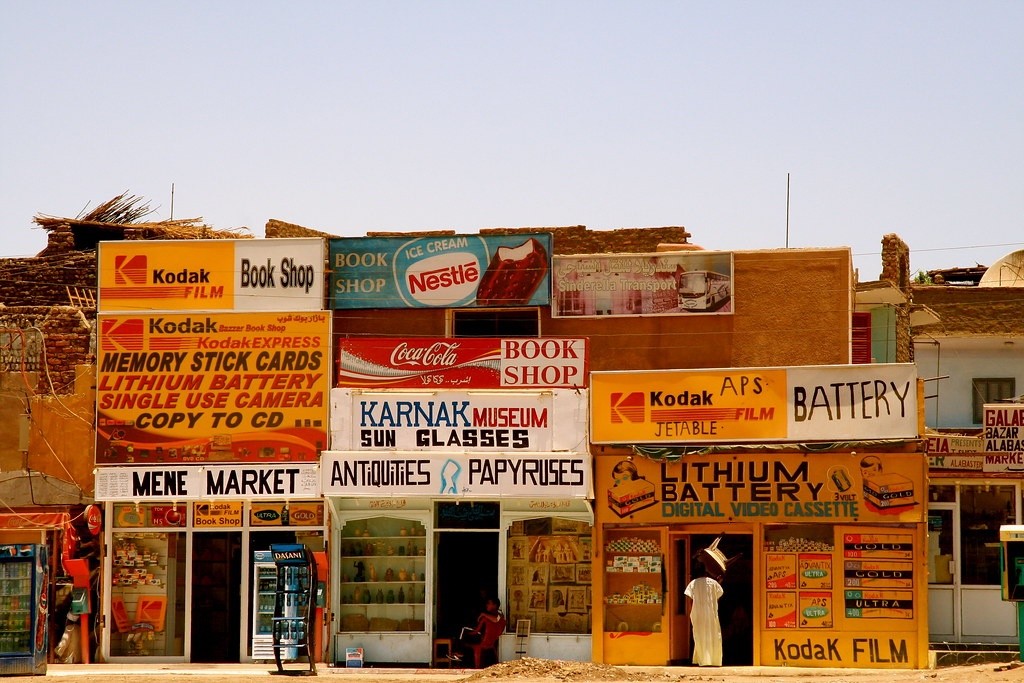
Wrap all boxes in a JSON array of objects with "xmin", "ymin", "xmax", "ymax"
[
  {"xmin": 340, "ymin": 535, "xmax": 426, "ymax": 634},
  {"xmin": 268, "ymin": 545, "xmax": 318, "ymax": 676}
]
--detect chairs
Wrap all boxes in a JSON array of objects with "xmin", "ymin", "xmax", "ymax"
[{"xmin": 456, "ymin": 618, "xmax": 507, "ymax": 669}]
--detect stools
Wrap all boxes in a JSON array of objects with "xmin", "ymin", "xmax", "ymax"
[{"xmin": 433, "ymin": 638, "xmax": 460, "ymax": 669}]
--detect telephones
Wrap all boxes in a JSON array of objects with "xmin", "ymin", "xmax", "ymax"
[{"xmin": 1013, "ymin": 556, "xmax": 1024, "ymax": 588}]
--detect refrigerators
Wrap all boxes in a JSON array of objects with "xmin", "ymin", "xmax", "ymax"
[
  {"xmin": 252, "ymin": 544, "xmax": 312, "ymax": 659},
  {"xmin": 0, "ymin": 544, "xmax": 47, "ymax": 675}
]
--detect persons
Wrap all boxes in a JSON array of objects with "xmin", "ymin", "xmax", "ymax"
[
  {"xmin": 684, "ymin": 574, "xmax": 724, "ymax": 667},
  {"xmin": 446, "ymin": 598, "xmax": 502, "ymax": 661}
]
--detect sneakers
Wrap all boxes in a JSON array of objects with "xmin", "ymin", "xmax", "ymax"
[{"xmin": 446, "ymin": 653, "xmax": 464, "ymax": 661}]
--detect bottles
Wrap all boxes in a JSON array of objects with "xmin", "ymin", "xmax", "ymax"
[
  {"xmin": 0, "ymin": 563, "xmax": 31, "ymax": 652},
  {"xmin": 36, "ymin": 566, "xmax": 49, "ymax": 650},
  {"xmin": 259, "ymin": 567, "xmax": 308, "ymax": 640}
]
